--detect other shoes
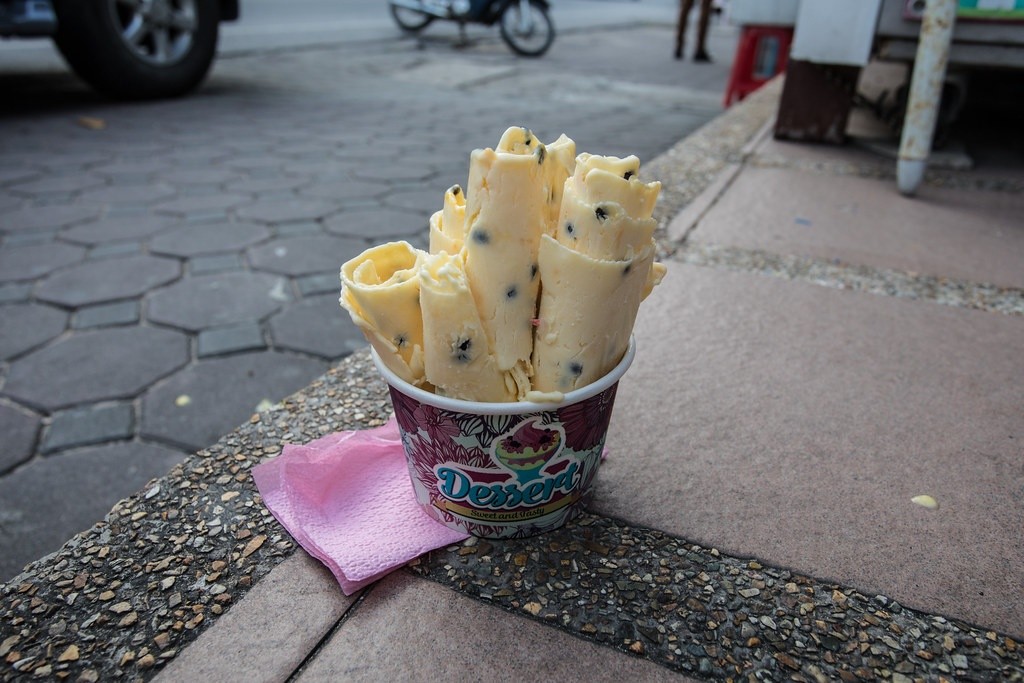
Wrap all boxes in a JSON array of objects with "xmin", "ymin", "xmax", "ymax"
[
  {"xmin": 675, "ymin": 52, "xmax": 681, "ymax": 58},
  {"xmin": 694, "ymin": 49, "xmax": 711, "ymax": 62}
]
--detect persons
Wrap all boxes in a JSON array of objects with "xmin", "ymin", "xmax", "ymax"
[{"xmin": 674, "ymin": 0, "xmax": 713, "ymax": 62}]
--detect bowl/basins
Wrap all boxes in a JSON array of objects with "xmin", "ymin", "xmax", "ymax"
[{"xmin": 370, "ymin": 331, "xmax": 636, "ymax": 540}]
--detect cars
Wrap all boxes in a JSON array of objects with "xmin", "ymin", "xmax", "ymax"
[{"xmin": 0, "ymin": 0, "xmax": 240, "ymax": 101}]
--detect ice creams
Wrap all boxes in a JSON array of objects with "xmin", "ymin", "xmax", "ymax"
[{"xmin": 336, "ymin": 125, "xmax": 670, "ymax": 540}]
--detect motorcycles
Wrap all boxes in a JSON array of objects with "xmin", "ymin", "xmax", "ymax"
[{"xmin": 389, "ymin": 1, "xmax": 555, "ymax": 59}]
